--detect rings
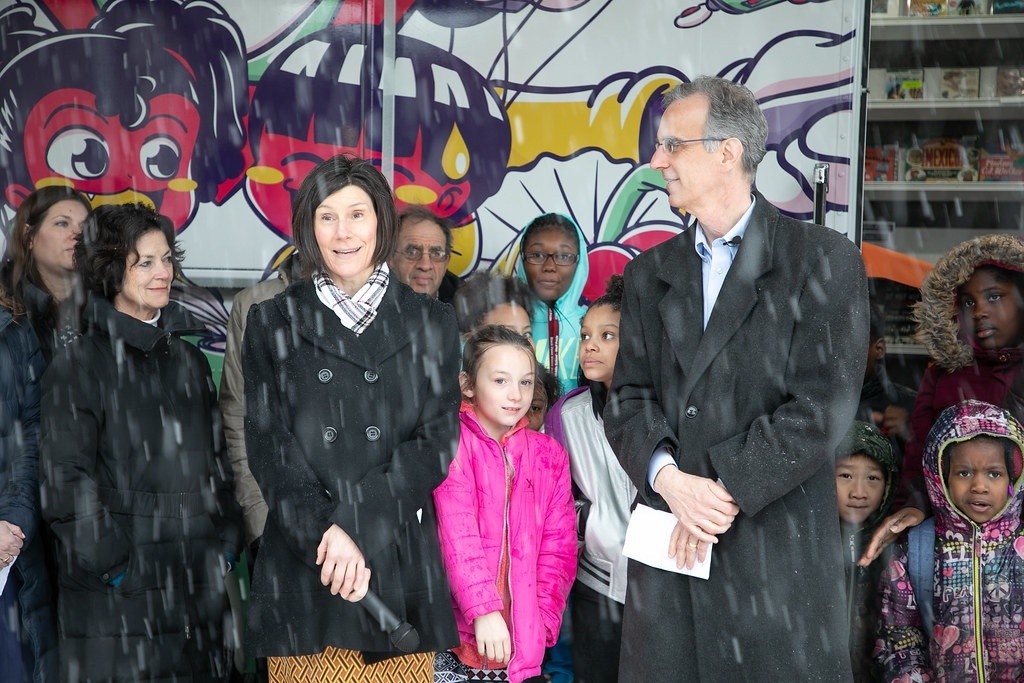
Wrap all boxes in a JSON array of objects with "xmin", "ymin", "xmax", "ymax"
[
  {"xmin": 688, "ymin": 542, "xmax": 696, "ymax": 548},
  {"xmin": 4, "ymin": 555, "xmax": 10, "ymax": 562}
]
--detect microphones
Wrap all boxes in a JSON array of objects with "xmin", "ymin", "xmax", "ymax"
[{"xmin": 360, "ymin": 588, "xmax": 419, "ymax": 654}]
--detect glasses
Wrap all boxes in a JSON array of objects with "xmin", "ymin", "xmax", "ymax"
[
  {"xmin": 654, "ymin": 133, "xmax": 727, "ymax": 155},
  {"xmin": 392, "ymin": 244, "xmax": 447, "ymax": 263},
  {"xmin": 521, "ymin": 251, "xmax": 580, "ymax": 266}
]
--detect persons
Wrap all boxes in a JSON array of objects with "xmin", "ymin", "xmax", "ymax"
[
  {"xmin": 433, "ymin": 326, "xmax": 578, "ymax": 683},
  {"xmin": 835, "ymin": 421, "xmax": 900, "ymax": 683},
  {"xmin": 941, "ymin": 72, "xmax": 976, "ymax": 98},
  {"xmin": 0, "ymin": 185, "xmax": 94, "ymax": 356},
  {"xmin": 858, "ymin": 234, "xmax": 1024, "ymax": 568},
  {"xmin": 885, "ymin": 74, "xmax": 905, "ymax": 99},
  {"xmin": 870, "ymin": 400, "xmax": 1024, "ymax": 683},
  {"xmin": 244, "ymin": 155, "xmax": 462, "ymax": 683},
  {"xmin": 455, "ymin": 213, "xmax": 594, "ymax": 537},
  {"xmin": 998, "ymin": 67, "xmax": 1024, "ymax": 96},
  {"xmin": 544, "ymin": 275, "xmax": 637, "ymax": 683},
  {"xmin": 0, "ymin": 284, "xmax": 59, "ymax": 683},
  {"xmin": 217, "ymin": 254, "xmax": 300, "ymax": 547},
  {"xmin": 386, "ymin": 208, "xmax": 464, "ymax": 304},
  {"xmin": 602, "ymin": 77, "xmax": 871, "ymax": 683},
  {"xmin": 855, "ymin": 305, "xmax": 918, "ymax": 451},
  {"xmin": 38, "ymin": 202, "xmax": 246, "ymax": 683}
]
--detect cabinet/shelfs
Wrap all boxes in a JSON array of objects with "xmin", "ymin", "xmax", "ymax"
[{"xmin": 859, "ymin": 0, "xmax": 1024, "ymax": 264}]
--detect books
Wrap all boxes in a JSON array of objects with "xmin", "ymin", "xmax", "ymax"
[{"xmin": 865, "ymin": 0, "xmax": 1024, "ymax": 342}]
